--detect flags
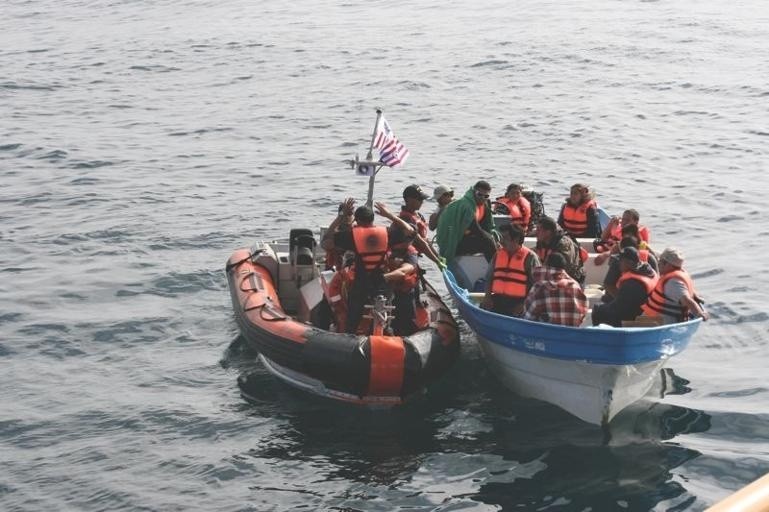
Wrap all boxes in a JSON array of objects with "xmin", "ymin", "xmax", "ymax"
[{"xmin": 373, "ymin": 116, "xmax": 407, "ymax": 169}]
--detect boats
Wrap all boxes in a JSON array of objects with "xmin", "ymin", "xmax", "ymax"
[
  {"xmin": 424, "ymin": 204, "xmax": 707, "ymax": 428},
  {"xmin": 227, "ymin": 226, "xmax": 458, "ymax": 411}
]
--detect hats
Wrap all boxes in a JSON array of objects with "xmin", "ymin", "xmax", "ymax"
[
  {"xmin": 654, "ymin": 247, "xmax": 685, "ymax": 268},
  {"xmin": 541, "ymin": 252, "xmax": 567, "ymax": 268},
  {"xmin": 403, "ymin": 184, "xmax": 430, "ymax": 201},
  {"xmin": 610, "ymin": 247, "xmax": 638, "ymax": 261},
  {"xmin": 430, "ymin": 186, "xmax": 455, "ymax": 199},
  {"xmin": 351, "ymin": 206, "xmax": 374, "ymax": 224}
]
